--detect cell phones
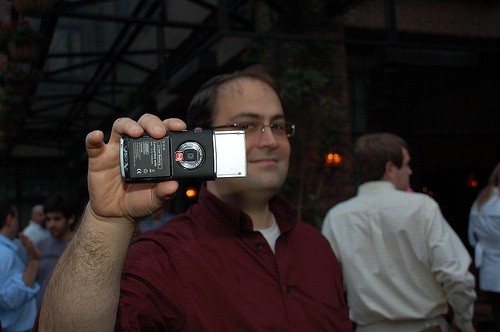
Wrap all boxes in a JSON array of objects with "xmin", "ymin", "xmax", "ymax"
[{"xmin": 119, "ymin": 130, "xmax": 247, "ymax": 182}]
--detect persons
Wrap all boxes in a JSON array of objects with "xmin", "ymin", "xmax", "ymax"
[
  {"xmin": 0, "ymin": 194, "xmax": 176, "ymax": 332},
  {"xmin": 320, "ymin": 133, "xmax": 476, "ymax": 332},
  {"xmin": 468, "ymin": 163, "xmax": 500, "ymax": 332},
  {"xmin": 39, "ymin": 63, "xmax": 357, "ymax": 332}
]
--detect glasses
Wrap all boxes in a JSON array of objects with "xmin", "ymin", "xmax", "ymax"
[{"xmin": 204, "ymin": 120, "xmax": 296, "ymax": 138}]
